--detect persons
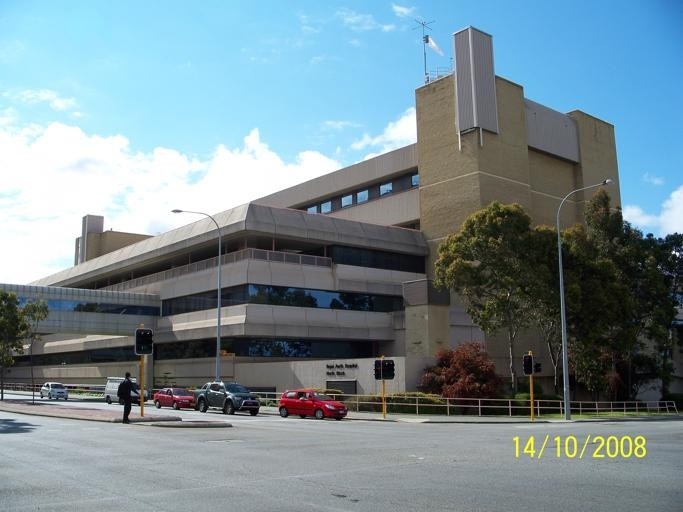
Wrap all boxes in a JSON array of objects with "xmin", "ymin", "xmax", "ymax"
[{"xmin": 116, "ymin": 371, "xmax": 140, "ymax": 424}]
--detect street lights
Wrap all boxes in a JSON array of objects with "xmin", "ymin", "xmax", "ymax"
[
  {"xmin": 171, "ymin": 209, "xmax": 221, "ymax": 380},
  {"xmin": 557, "ymin": 178, "xmax": 615, "ymax": 420}
]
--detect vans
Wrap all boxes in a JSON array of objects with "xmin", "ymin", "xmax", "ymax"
[{"xmin": 104, "ymin": 376, "xmax": 149, "ymax": 406}]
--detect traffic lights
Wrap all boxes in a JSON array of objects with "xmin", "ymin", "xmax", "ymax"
[
  {"xmin": 522, "ymin": 354, "xmax": 533, "ymax": 374},
  {"xmin": 138, "ymin": 330, "xmax": 152, "ymax": 355},
  {"xmin": 372, "ymin": 362, "xmax": 380, "ymax": 377},
  {"xmin": 384, "ymin": 362, "xmax": 395, "ymax": 379}
]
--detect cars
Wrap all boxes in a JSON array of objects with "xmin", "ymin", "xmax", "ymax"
[
  {"xmin": 40, "ymin": 382, "xmax": 69, "ymax": 400},
  {"xmin": 278, "ymin": 389, "xmax": 348, "ymax": 419},
  {"xmin": 153, "ymin": 381, "xmax": 261, "ymax": 416}
]
